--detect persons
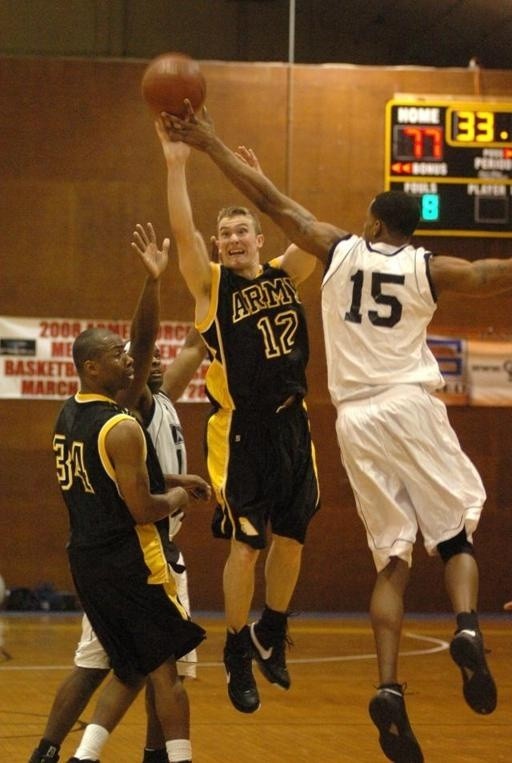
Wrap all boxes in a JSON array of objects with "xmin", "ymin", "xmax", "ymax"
[
  {"xmin": 160, "ymin": 98, "xmax": 512, "ymax": 763},
  {"xmin": 155, "ymin": 117, "xmax": 317, "ymax": 715}
]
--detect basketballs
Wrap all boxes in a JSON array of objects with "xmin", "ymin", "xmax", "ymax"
[{"xmin": 142, "ymin": 53, "xmax": 206, "ymax": 118}]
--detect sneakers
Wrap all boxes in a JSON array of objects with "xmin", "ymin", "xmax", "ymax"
[
  {"xmin": 369, "ymin": 684, "xmax": 424, "ymax": 763},
  {"xmin": 449, "ymin": 627, "xmax": 496, "ymax": 715},
  {"xmin": 224, "ymin": 618, "xmax": 290, "ymax": 714}
]
